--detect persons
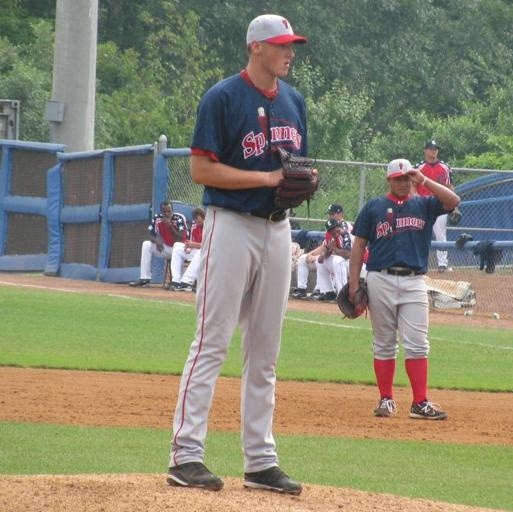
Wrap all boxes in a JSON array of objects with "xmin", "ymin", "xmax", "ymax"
[
  {"xmin": 165, "ymin": 12, "xmax": 319, "ymax": 496},
  {"xmin": 290, "ymin": 204, "xmax": 352, "ymax": 300},
  {"xmin": 317, "ymin": 218, "xmax": 352, "ymax": 304},
  {"xmin": 346, "ymin": 159, "xmax": 462, "ymax": 420},
  {"xmin": 129, "ymin": 201, "xmax": 188, "ymax": 291},
  {"xmin": 169, "ymin": 207, "xmax": 205, "ymax": 293},
  {"xmin": 413, "ymin": 139, "xmax": 457, "ymax": 273}
]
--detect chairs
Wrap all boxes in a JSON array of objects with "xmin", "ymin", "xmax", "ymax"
[{"xmin": 165, "ymin": 229, "xmax": 326, "ymax": 300}]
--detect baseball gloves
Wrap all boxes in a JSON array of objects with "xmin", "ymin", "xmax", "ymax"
[
  {"xmin": 457, "ymin": 233, "xmax": 474, "ymax": 249},
  {"xmin": 273, "ymin": 147, "xmax": 319, "ymax": 209},
  {"xmin": 336, "ymin": 277, "xmax": 368, "ymax": 319},
  {"xmin": 449, "ymin": 208, "xmax": 462, "ymax": 224}
]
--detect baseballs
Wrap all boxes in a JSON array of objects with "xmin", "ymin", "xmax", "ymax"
[
  {"xmin": 465, "ymin": 311, "xmax": 472, "ymax": 316},
  {"xmin": 492, "ymin": 313, "xmax": 500, "ymax": 319}
]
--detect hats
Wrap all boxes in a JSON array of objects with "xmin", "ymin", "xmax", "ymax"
[
  {"xmin": 244, "ymin": 13, "xmax": 307, "ymax": 48},
  {"xmin": 323, "ymin": 202, "xmax": 344, "ymax": 216},
  {"xmin": 384, "ymin": 158, "xmax": 418, "ymax": 180},
  {"xmin": 422, "ymin": 138, "xmax": 441, "ymax": 151},
  {"xmin": 324, "ymin": 218, "xmax": 341, "ymax": 232}
]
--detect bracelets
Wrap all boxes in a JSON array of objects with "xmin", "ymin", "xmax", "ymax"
[{"xmin": 420, "ymin": 176, "xmax": 426, "ymax": 186}]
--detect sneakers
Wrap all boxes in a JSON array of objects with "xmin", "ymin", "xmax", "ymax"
[
  {"xmin": 164, "ymin": 460, "xmax": 225, "ymax": 492},
  {"xmin": 240, "ymin": 464, "xmax": 304, "ymax": 497},
  {"xmin": 372, "ymin": 396, "xmax": 398, "ymax": 418},
  {"xmin": 406, "ymin": 397, "xmax": 450, "ymax": 422},
  {"xmin": 127, "ymin": 279, "xmax": 149, "ymax": 288},
  {"xmin": 289, "ymin": 288, "xmax": 337, "ymax": 303},
  {"xmin": 161, "ymin": 281, "xmax": 192, "ymax": 292},
  {"xmin": 438, "ymin": 266, "xmax": 447, "ymax": 274}
]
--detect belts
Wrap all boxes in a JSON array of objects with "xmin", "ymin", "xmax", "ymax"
[
  {"xmin": 208, "ymin": 202, "xmax": 293, "ymax": 224},
  {"xmin": 377, "ymin": 265, "xmax": 425, "ymax": 277}
]
